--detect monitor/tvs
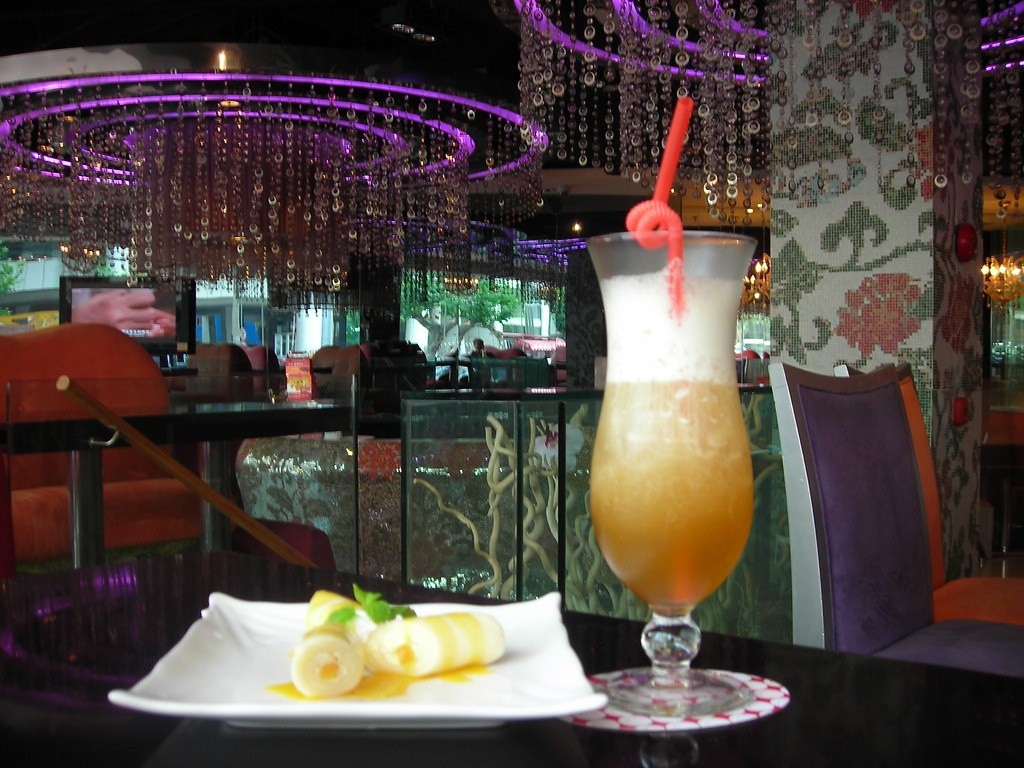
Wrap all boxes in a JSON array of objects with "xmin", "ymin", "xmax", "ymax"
[{"xmin": 60, "ymin": 276, "xmax": 198, "ymax": 354}]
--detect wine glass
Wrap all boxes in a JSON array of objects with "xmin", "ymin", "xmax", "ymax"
[{"xmin": 561, "ymin": 231, "xmax": 791, "ymax": 734}]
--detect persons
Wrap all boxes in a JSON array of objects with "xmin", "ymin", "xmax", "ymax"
[
  {"xmin": 72, "ymin": 287, "xmax": 176, "ymax": 339},
  {"xmin": 470, "ymin": 339, "xmax": 495, "ymax": 384}
]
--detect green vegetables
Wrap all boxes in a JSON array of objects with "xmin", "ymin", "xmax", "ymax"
[{"xmin": 323, "ymin": 582, "xmax": 418, "ymax": 626}]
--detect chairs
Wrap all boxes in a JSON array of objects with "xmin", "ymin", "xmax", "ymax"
[{"xmin": 0, "ymin": 324, "xmax": 1024, "ymax": 677}]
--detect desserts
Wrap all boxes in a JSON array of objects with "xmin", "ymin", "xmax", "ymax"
[{"xmin": 290, "ymin": 587, "xmax": 507, "ymax": 699}]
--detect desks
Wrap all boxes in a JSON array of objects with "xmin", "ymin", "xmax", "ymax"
[{"xmin": 1, "ymin": 544, "xmax": 1023, "ymax": 768}]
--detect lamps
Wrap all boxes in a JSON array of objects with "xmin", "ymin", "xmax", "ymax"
[
  {"xmin": 981, "ymin": 202, "xmax": 1024, "ymax": 315},
  {"xmin": 732, "ymin": 208, "xmax": 772, "ymax": 311}
]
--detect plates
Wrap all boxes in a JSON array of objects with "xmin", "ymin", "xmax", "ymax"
[{"xmin": 109, "ymin": 591, "xmax": 612, "ymax": 730}]
